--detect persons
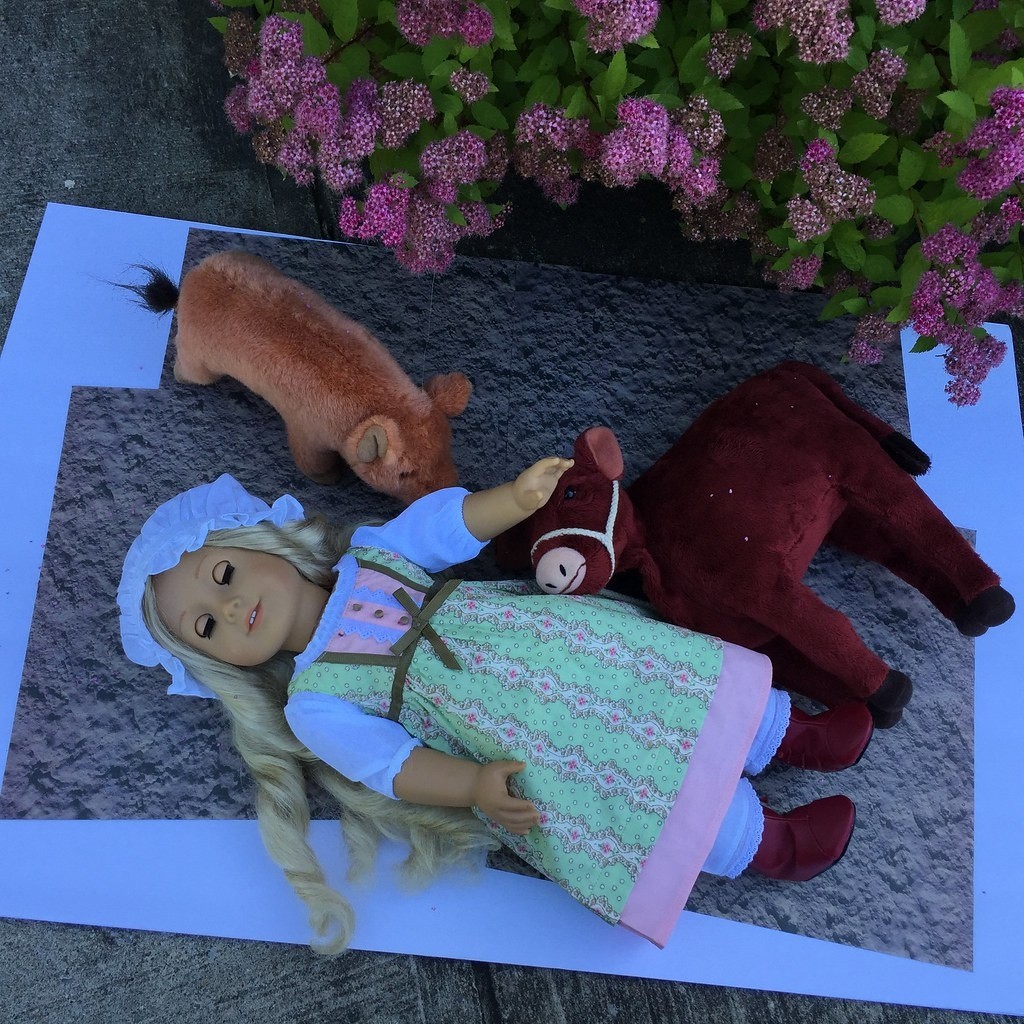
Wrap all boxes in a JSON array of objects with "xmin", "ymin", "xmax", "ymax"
[{"xmin": 121, "ymin": 479, "xmax": 875, "ymax": 937}]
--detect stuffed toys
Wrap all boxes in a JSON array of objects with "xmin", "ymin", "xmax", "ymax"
[
  {"xmin": 490, "ymin": 362, "xmax": 1019, "ymax": 730},
  {"xmin": 137, "ymin": 248, "xmax": 471, "ymax": 507}
]
